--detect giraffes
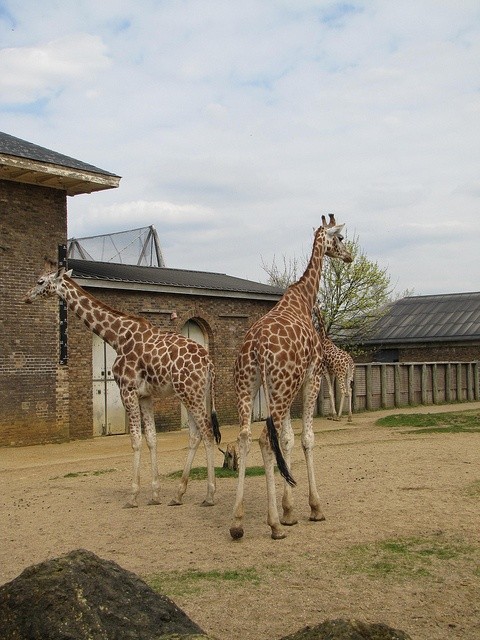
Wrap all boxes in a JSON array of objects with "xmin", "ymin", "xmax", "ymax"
[
  {"xmin": 228, "ymin": 213, "xmax": 354, "ymax": 541},
  {"xmin": 311, "ymin": 303, "xmax": 354, "ymax": 422},
  {"xmin": 23, "ymin": 257, "xmax": 223, "ymax": 509}
]
